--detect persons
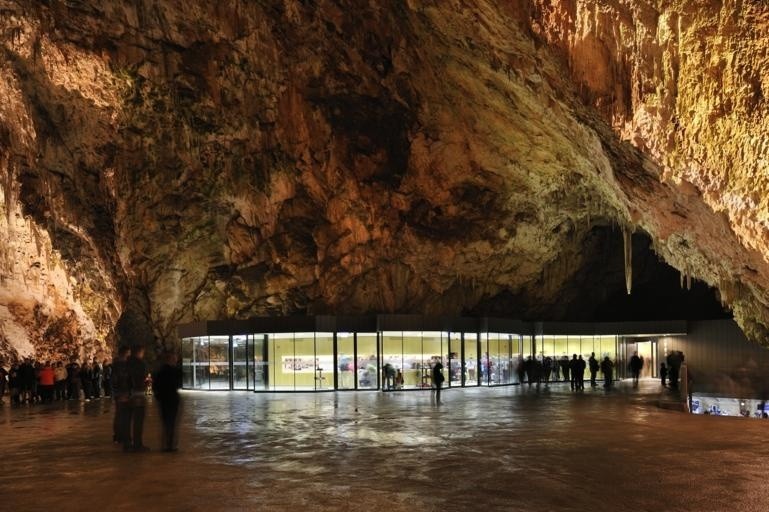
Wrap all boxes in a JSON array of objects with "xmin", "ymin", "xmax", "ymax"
[
  {"xmin": 359, "ymin": 363, "xmax": 404, "ymax": 389},
  {"xmin": 114, "ymin": 345, "xmax": 132, "ymax": 443},
  {"xmin": 419, "ymin": 350, "xmax": 684, "ymax": 406},
  {"xmin": 0, "ymin": 355, "xmax": 113, "ymax": 405},
  {"xmin": 122, "ymin": 346, "xmax": 150, "ymax": 451},
  {"xmin": 152, "ymin": 353, "xmax": 183, "ymax": 453}
]
